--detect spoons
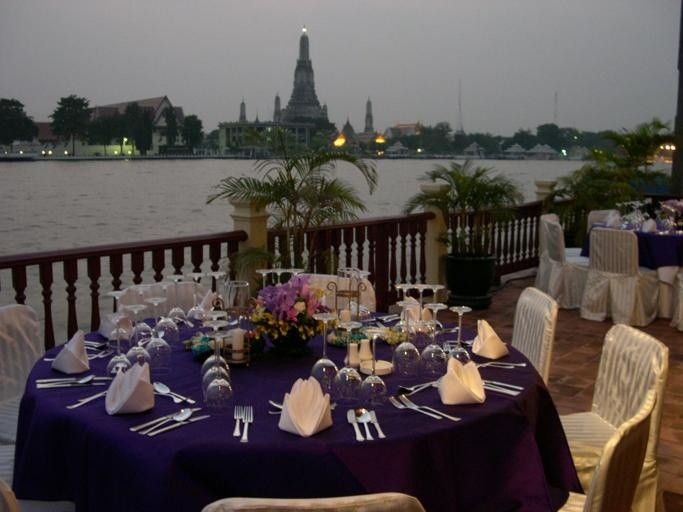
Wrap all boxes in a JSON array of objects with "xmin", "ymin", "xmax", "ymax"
[
  {"xmin": 356, "ymin": 408, "xmax": 375, "ymax": 441},
  {"xmin": 138, "ymin": 407, "xmax": 192, "ymax": 435},
  {"xmin": 152, "ymin": 381, "xmax": 197, "ymax": 404},
  {"xmin": 397, "ymin": 381, "xmax": 436, "ymax": 392},
  {"xmin": 34, "ymin": 374, "xmax": 94, "ymax": 384},
  {"xmin": 85, "ymin": 343, "xmax": 107, "ymax": 349}
]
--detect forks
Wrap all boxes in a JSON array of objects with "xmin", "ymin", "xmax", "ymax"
[
  {"xmin": 388, "ymin": 396, "xmax": 442, "ymax": 421},
  {"xmin": 346, "ymin": 409, "xmax": 364, "ymax": 442},
  {"xmin": 233, "ymin": 405, "xmax": 244, "ymax": 436},
  {"xmin": 398, "ymin": 393, "xmax": 463, "ymax": 422},
  {"xmin": 239, "ymin": 406, "xmax": 253, "ymax": 443}
]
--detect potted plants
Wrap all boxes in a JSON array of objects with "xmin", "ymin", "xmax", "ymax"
[{"xmin": 402, "ymin": 155, "xmax": 526, "ymax": 310}]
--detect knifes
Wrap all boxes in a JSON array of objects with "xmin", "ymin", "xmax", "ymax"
[
  {"xmin": 370, "ymin": 411, "xmax": 386, "ymax": 439},
  {"xmin": 37, "ymin": 376, "xmax": 113, "ymax": 390},
  {"xmin": 147, "ymin": 415, "xmax": 210, "ymax": 437},
  {"xmin": 483, "ymin": 380, "xmax": 525, "ymax": 391},
  {"xmin": 86, "ymin": 340, "xmax": 123, "ymax": 350},
  {"xmin": 128, "ymin": 407, "xmax": 201, "ymax": 432}
]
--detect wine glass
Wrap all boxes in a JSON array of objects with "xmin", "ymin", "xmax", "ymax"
[
  {"xmin": 397, "ymin": 284, "xmax": 418, "ymax": 344},
  {"xmin": 358, "ymin": 326, "xmax": 389, "ymax": 407},
  {"xmin": 167, "ymin": 275, "xmax": 188, "ymax": 329},
  {"xmin": 124, "ymin": 304, "xmax": 153, "ymax": 368},
  {"xmin": 391, "ymin": 302, "xmax": 421, "ymax": 379},
  {"xmin": 186, "ymin": 272, "xmax": 206, "ymax": 328},
  {"xmin": 332, "ymin": 321, "xmax": 363, "ymax": 403},
  {"xmin": 106, "ymin": 291, "xmax": 130, "ymax": 351},
  {"xmin": 201, "ymin": 320, "xmax": 231, "ymax": 402},
  {"xmin": 131, "ymin": 285, "xmax": 152, "ymax": 345},
  {"xmin": 355, "ymin": 270, "xmax": 372, "ymax": 326},
  {"xmin": 154, "ymin": 281, "xmax": 180, "ymax": 344},
  {"xmin": 412, "ymin": 284, "xmax": 431, "ymax": 338},
  {"xmin": 108, "ymin": 313, "xmax": 132, "ymax": 380},
  {"xmin": 447, "ymin": 306, "xmax": 471, "ymax": 365},
  {"xmin": 345, "ymin": 269, "xmax": 361, "ymax": 318},
  {"xmin": 426, "ymin": 284, "xmax": 446, "ymax": 342},
  {"xmin": 144, "ymin": 296, "xmax": 173, "ymax": 365},
  {"xmin": 200, "ymin": 311, "xmax": 230, "ymax": 375},
  {"xmin": 420, "ymin": 303, "xmax": 449, "ymax": 378},
  {"xmin": 206, "ymin": 332, "xmax": 234, "ymax": 412},
  {"xmin": 309, "ymin": 312, "xmax": 339, "ymax": 403}
]
